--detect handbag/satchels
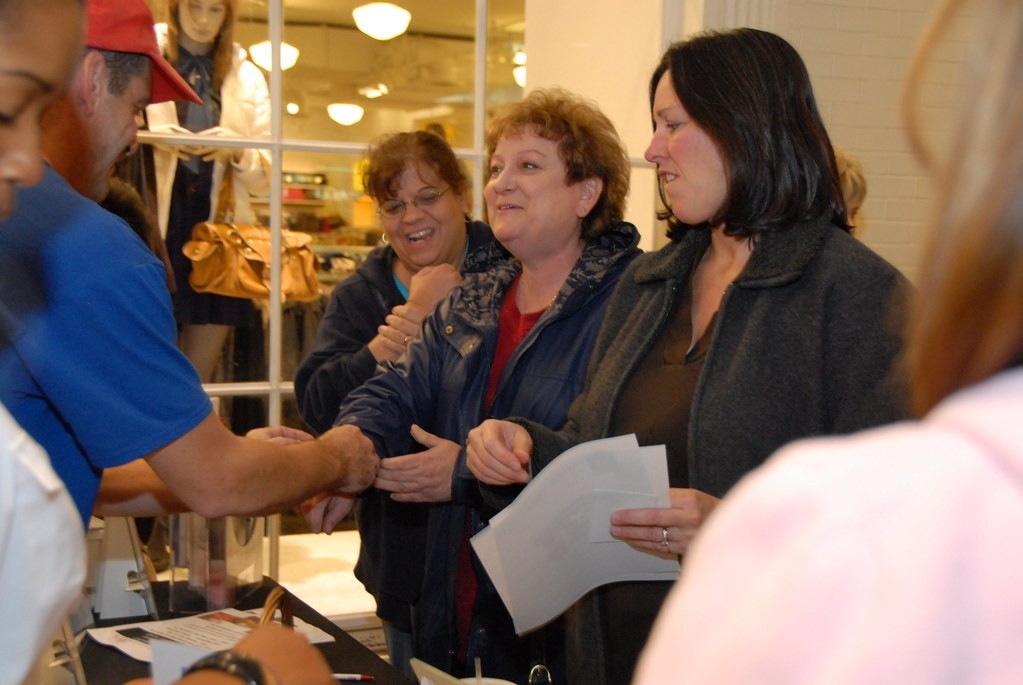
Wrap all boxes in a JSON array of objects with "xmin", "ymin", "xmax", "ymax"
[{"xmin": 183, "ymin": 146, "xmax": 320, "ymax": 304}]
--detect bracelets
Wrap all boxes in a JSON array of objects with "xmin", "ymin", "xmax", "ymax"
[{"xmin": 183, "ymin": 649, "xmax": 267, "ymax": 685}]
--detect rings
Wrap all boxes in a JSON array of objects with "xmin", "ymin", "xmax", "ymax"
[
  {"xmin": 403, "ymin": 335, "xmax": 408, "ymax": 347},
  {"xmin": 662, "ymin": 540, "xmax": 671, "ymax": 555},
  {"xmin": 660, "ymin": 528, "xmax": 668, "ymax": 545}
]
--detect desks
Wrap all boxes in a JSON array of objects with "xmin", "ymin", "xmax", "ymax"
[{"xmin": 73, "ymin": 574, "xmax": 422, "ymax": 685}]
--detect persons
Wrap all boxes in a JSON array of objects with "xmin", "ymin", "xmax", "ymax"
[
  {"xmin": 116, "ymin": 0, "xmax": 272, "ymax": 583},
  {"xmin": 0, "ymin": 0, "xmax": 1022, "ymax": 685}
]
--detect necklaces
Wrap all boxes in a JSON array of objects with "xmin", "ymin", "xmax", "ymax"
[{"xmin": 521, "ymin": 281, "xmax": 556, "ymax": 315}]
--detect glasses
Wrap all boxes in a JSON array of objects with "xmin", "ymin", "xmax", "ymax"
[{"xmin": 378, "ymin": 185, "xmax": 452, "ymax": 219}]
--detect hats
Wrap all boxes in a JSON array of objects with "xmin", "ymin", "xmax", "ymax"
[{"xmin": 85, "ymin": 0, "xmax": 204, "ymax": 105}]
